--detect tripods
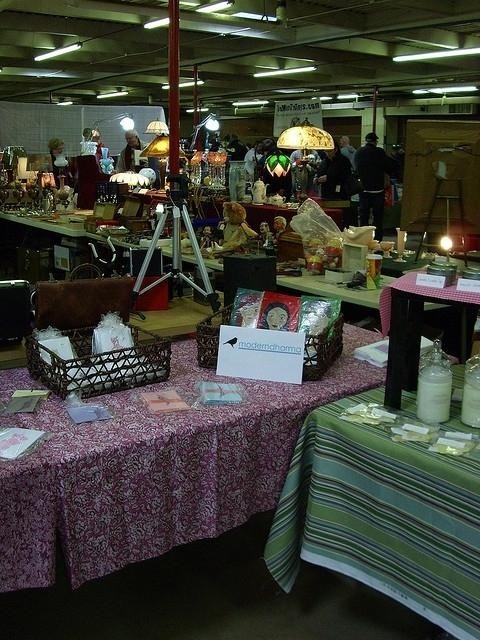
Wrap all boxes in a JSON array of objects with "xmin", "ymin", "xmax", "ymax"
[{"xmin": 128, "ymin": 0, "xmax": 220, "ymax": 316}]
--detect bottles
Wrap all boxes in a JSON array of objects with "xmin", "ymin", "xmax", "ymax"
[
  {"xmin": 415, "ymin": 337, "xmax": 452, "ymax": 424},
  {"xmin": 461, "ymin": 353, "xmax": 480, "ymax": 429}
]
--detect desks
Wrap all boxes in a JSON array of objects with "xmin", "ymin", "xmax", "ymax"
[
  {"xmin": 1, "ymin": 199, "xmax": 478, "ymax": 363},
  {"xmin": 1, "ymin": 324, "xmax": 477, "ymax": 640}
]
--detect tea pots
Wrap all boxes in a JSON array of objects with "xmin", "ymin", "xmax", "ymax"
[{"xmin": 249, "ymin": 178, "xmax": 270, "ymax": 206}]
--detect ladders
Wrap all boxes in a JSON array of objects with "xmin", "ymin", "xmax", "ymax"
[{"xmin": 415, "ymin": 179, "xmax": 468, "ymax": 267}]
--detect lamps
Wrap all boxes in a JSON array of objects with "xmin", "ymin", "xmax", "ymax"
[
  {"xmin": 138, "ymin": 117, "xmax": 172, "ymax": 194},
  {"xmin": 79, "ymin": 114, "xmax": 135, "ymax": 156},
  {"xmin": 190, "ymin": 113, "xmax": 220, "ymax": 151},
  {"xmin": 254, "ymin": 118, "xmax": 335, "ymax": 205}
]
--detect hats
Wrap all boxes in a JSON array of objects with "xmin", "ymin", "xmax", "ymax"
[{"xmin": 366, "ymin": 133, "xmax": 379, "ymax": 142}]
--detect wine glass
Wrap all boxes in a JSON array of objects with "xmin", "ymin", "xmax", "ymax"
[
  {"xmin": 367, "ymin": 231, "xmax": 408, "ymax": 263},
  {"xmin": 43, "ymin": 199, "xmax": 50, "ymax": 218}
]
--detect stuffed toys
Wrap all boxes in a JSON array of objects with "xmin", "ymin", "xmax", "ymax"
[{"xmin": 202, "ymin": 201, "xmax": 287, "ymax": 252}]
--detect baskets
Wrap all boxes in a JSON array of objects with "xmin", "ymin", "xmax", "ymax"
[
  {"xmin": 25, "ymin": 325, "xmax": 172, "ymax": 400},
  {"xmin": 195, "ymin": 301, "xmax": 344, "ymax": 381}
]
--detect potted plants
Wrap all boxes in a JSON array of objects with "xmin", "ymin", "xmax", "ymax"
[{"xmin": 48, "ymin": 137, "xmax": 64, "ymax": 155}]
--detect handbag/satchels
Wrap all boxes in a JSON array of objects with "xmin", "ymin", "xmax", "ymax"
[
  {"xmin": 384, "ymin": 174, "xmax": 396, "ymax": 208},
  {"xmin": 36, "ymin": 263, "xmax": 134, "ymax": 325}
]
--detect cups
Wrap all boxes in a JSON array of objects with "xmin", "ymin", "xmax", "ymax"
[{"xmin": 366, "ymin": 252, "xmax": 383, "ymax": 290}]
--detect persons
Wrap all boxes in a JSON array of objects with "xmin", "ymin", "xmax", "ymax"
[
  {"xmin": 302, "ymin": 299, "xmax": 332, "ymax": 334},
  {"xmin": 224, "ymin": 132, "xmax": 401, "ymax": 243},
  {"xmin": 42, "ymin": 137, "xmax": 73, "ymax": 187},
  {"xmin": 260, "ymin": 303, "xmax": 289, "ymax": 331},
  {"xmin": 235, "ymin": 295, "xmax": 260, "ymax": 328},
  {"xmin": 115, "ymin": 130, "xmax": 150, "ymax": 171}
]
